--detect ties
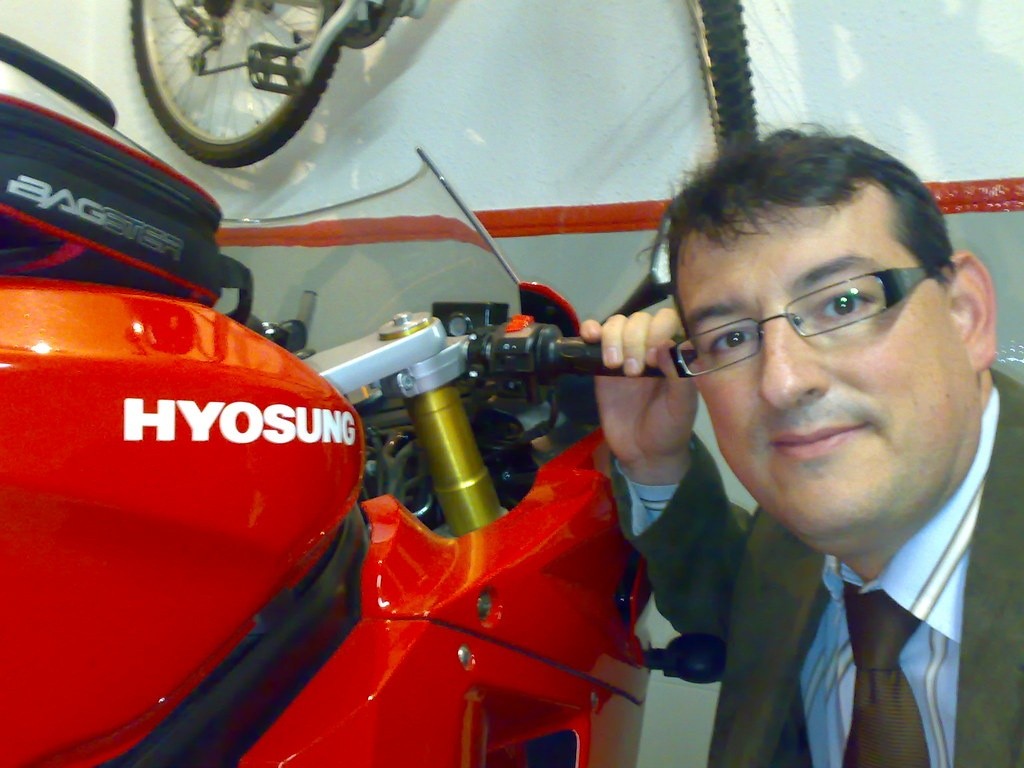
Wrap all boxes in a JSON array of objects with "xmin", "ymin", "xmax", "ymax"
[{"xmin": 843, "ymin": 581, "xmax": 930, "ymax": 768}]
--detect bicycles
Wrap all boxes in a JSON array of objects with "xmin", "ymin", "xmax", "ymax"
[{"xmin": 126, "ymin": 1, "xmax": 424, "ymax": 168}]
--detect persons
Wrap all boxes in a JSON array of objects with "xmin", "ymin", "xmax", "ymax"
[{"xmin": 581, "ymin": 121, "xmax": 1023, "ymax": 768}]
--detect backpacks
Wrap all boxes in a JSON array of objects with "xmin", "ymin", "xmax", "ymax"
[{"xmin": 0, "ymin": 31, "xmax": 253, "ymax": 326}]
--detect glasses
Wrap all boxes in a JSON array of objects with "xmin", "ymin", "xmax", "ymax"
[{"xmin": 675, "ymin": 265, "xmax": 931, "ymax": 376}]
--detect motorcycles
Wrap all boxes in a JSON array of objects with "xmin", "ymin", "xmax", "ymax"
[{"xmin": 0, "ymin": 31, "xmax": 684, "ymax": 768}]
